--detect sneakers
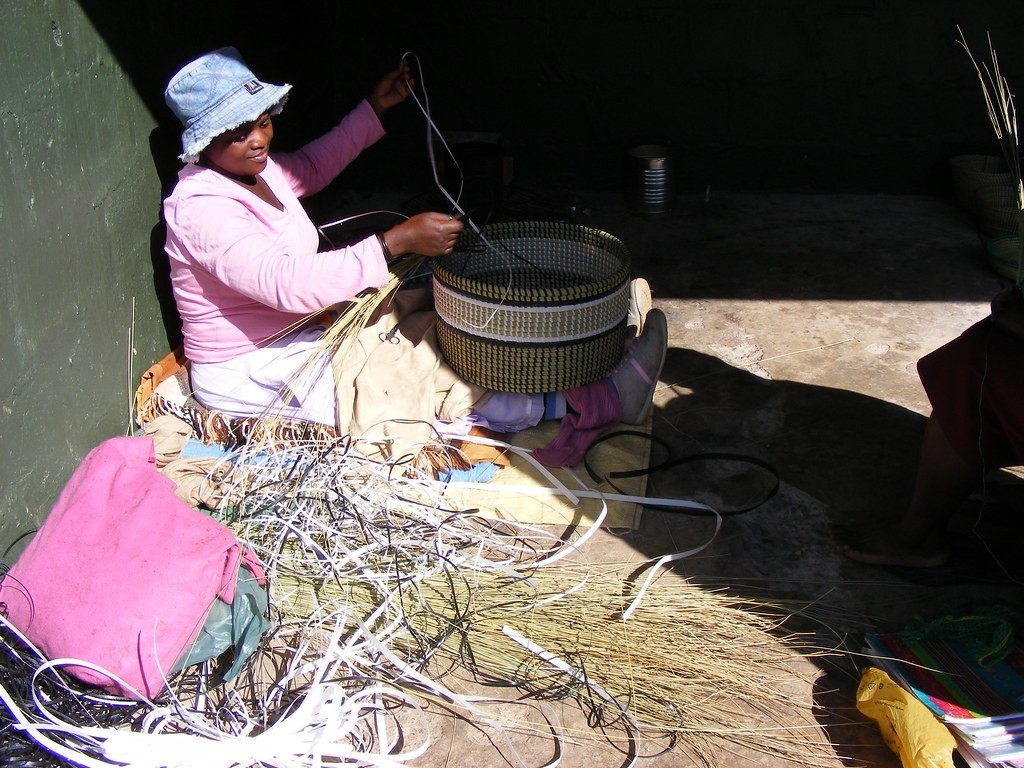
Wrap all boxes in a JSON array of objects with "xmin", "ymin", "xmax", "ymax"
[
  {"xmin": 624, "ymin": 277, "xmax": 652, "ymax": 358},
  {"xmin": 606, "ymin": 308, "xmax": 669, "ymax": 426}
]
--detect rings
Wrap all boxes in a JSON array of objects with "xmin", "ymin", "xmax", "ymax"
[{"xmin": 449, "ymin": 214, "xmax": 453, "ymax": 221}]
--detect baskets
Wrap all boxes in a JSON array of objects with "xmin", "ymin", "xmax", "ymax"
[{"xmin": 433, "ymin": 221, "xmax": 631, "ymax": 395}]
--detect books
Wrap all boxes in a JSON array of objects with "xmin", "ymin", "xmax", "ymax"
[{"xmin": 856, "ymin": 606, "xmax": 1024, "ymax": 768}]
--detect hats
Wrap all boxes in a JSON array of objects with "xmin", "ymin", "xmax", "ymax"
[{"xmin": 165, "ymin": 47, "xmax": 293, "ymax": 166}]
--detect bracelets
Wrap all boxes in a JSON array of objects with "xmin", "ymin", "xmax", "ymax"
[{"xmin": 377, "ymin": 234, "xmax": 395, "ymax": 263}]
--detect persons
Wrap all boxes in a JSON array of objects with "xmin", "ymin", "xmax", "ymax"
[
  {"xmin": 828, "ymin": 284, "xmax": 1024, "ymax": 568},
  {"xmin": 160, "ymin": 44, "xmax": 668, "ymax": 433}
]
